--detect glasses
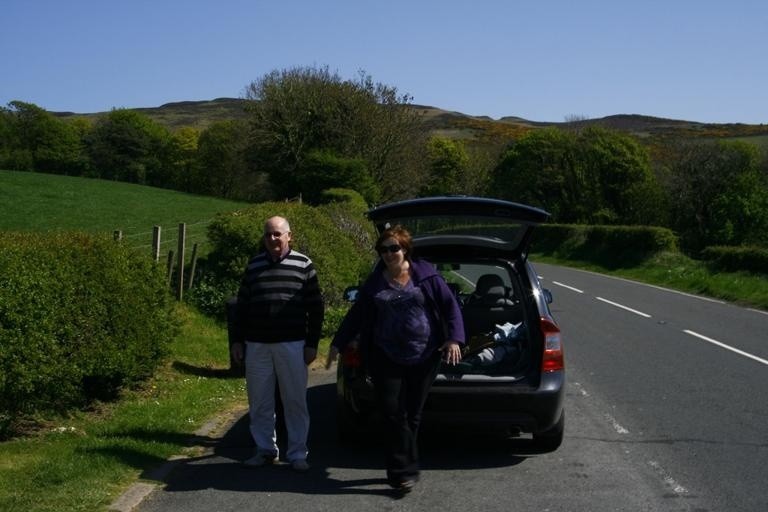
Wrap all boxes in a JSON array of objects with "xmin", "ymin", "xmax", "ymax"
[
  {"xmin": 264, "ymin": 231, "xmax": 289, "ymax": 239},
  {"xmin": 376, "ymin": 244, "xmax": 404, "ymax": 254}
]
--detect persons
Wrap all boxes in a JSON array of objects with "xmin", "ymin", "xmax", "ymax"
[
  {"xmin": 230, "ymin": 215, "xmax": 325, "ymax": 470},
  {"xmin": 325, "ymin": 224, "xmax": 465, "ymax": 494}
]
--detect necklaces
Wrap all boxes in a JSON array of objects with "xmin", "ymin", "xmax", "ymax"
[{"xmin": 390, "ymin": 272, "xmax": 404, "ymax": 288}]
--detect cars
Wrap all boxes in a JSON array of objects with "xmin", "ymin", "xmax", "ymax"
[{"xmin": 324, "ymin": 194, "xmax": 573, "ymax": 452}]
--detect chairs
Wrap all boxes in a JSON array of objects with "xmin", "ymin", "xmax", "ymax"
[{"xmin": 465, "ymin": 274, "xmax": 513, "ymax": 314}]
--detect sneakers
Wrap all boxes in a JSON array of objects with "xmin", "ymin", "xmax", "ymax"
[
  {"xmin": 292, "ymin": 461, "xmax": 309, "ymax": 471},
  {"xmin": 243, "ymin": 453, "xmax": 280, "ymax": 467},
  {"xmin": 388, "ymin": 473, "xmax": 418, "ymax": 495}
]
